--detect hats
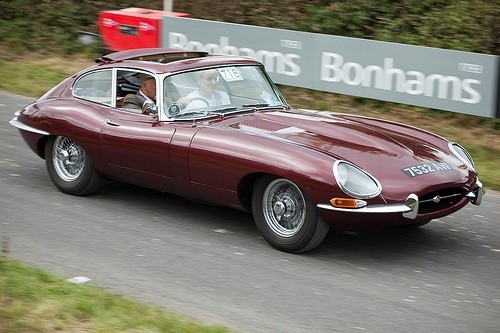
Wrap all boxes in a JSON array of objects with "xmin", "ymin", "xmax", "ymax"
[{"xmin": 140, "ymin": 69, "xmax": 154, "ymax": 82}]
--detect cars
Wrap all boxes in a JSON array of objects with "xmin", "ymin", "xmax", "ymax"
[{"xmin": 9, "ymin": 49, "xmax": 487, "ymax": 254}]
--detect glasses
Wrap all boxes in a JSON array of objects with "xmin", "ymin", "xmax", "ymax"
[{"xmin": 203, "ymin": 76, "xmax": 219, "ymax": 81}]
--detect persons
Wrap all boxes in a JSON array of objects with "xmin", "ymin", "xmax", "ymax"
[
  {"xmin": 121, "ymin": 73, "xmax": 187, "ymax": 117},
  {"xmin": 185, "ymin": 70, "xmax": 231, "ymax": 113}
]
